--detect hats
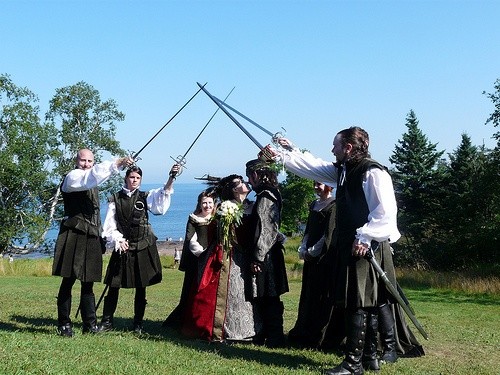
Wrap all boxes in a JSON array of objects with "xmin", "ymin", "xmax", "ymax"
[{"xmin": 246, "ymin": 159, "xmax": 269, "ymax": 176}]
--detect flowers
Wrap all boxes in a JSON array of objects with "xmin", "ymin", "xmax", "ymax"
[{"xmin": 209, "ymin": 200, "xmax": 251, "ymax": 254}]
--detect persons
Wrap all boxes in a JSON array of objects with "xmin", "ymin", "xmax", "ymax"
[
  {"xmin": 215, "ymin": 175, "xmax": 256, "ymax": 343},
  {"xmin": 290, "ymin": 180, "xmax": 336, "ymax": 347},
  {"xmin": 276, "ymin": 127, "xmax": 400, "ymax": 363},
  {"xmin": 51, "ymin": 149, "xmax": 134, "ymax": 338},
  {"xmin": 246, "ymin": 159, "xmax": 289, "ymax": 349},
  {"xmin": 266, "ymin": 129, "xmax": 397, "ymax": 375},
  {"xmin": 98, "ymin": 163, "xmax": 183, "ymax": 335},
  {"xmin": 177, "ymin": 191, "xmax": 224, "ymax": 342}
]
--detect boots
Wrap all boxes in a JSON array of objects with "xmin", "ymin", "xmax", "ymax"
[
  {"xmin": 98, "ymin": 296, "xmax": 118, "ymax": 332},
  {"xmin": 320, "ymin": 310, "xmax": 368, "ymax": 375},
  {"xmin": 81, "ymin": 294, "xmax": 100, "ymax": 334},
  {"xmin": 133, "ymin": 299, "xmax": 148, "ymax": 334},
  {"xmin": 55, "ymin": 295, "xmax": 75, "ymax": 337},
  {"xmin": 376, "ymin": 302, "xmax": 398, "ymax": 365},
  {"xmin": 362, "ymin": 305, "xmax": 381, "ymax": 371}
]
